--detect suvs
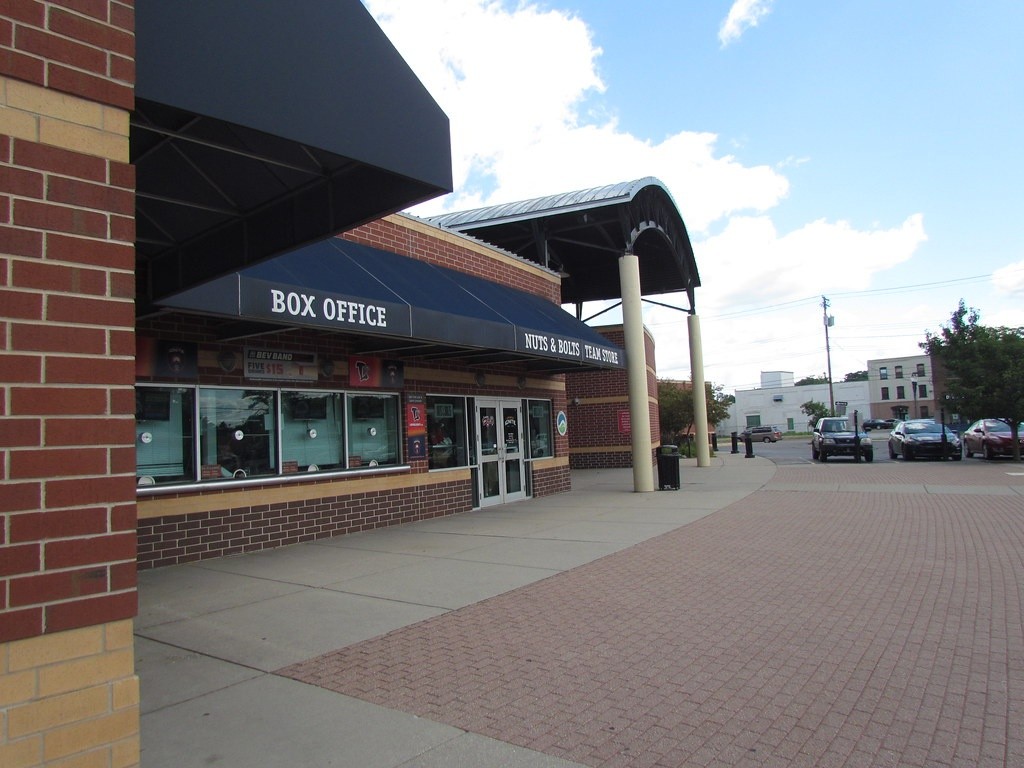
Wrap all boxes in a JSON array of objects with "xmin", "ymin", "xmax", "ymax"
[{"xmin": 811, "ymin": 416, "xmax": 874, "ymax": 461}]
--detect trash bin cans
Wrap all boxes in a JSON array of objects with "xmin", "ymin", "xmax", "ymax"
[{"xmin": 654, "ymin": 445, "xmax": 681, "ymax": 490}]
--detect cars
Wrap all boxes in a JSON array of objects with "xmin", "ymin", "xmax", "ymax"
[
  {"xmin": 888, "ymin": 419, "xmax": 963, "ymax": 460},
  {"xmin": 863, "ymin": 419, "xmax": 893, "ymax": 430},
  {"xmin": 963, "ymin": 418, "xmax": 1024, "ymax": 460},
  {"xmin": 737, "ymin": 425, "xmax": 783, "ymax": 443},
  {"xmin": 885, "ymin": 419, "xmax": 902, "ymax": 427}
]
